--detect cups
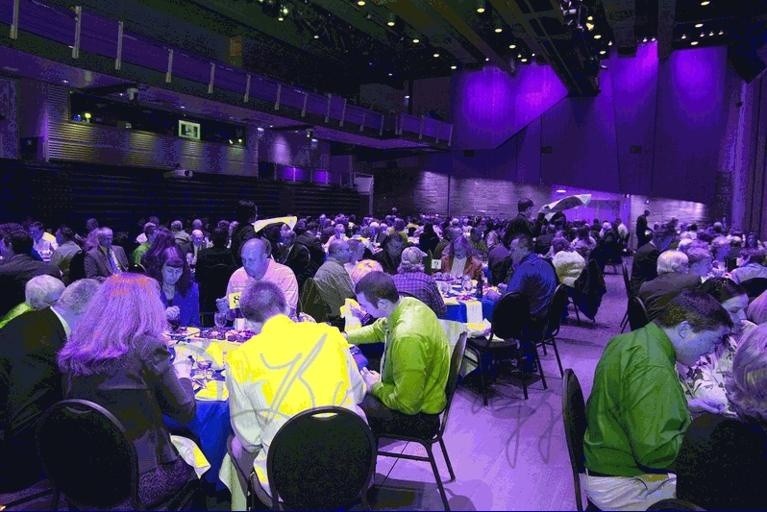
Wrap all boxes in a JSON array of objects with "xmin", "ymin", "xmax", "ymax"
[{"xmin": 233, "ymin": 318, "xmax": 246, "ymax": 332}]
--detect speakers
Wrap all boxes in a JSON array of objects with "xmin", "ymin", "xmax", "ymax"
[
  {"xmin": 464, "ymin": 149, "xmax": 474, "ymax": 158},
  {"xmin": 22, "ymin": 136, "xmax": 39, "ymax": 160},
  {"xmin": 543, "ymin": 146, "xmax": 553, "ymax": 154},
  {"xmin": 331, "ymin": 142, "xmax": 355, "ymax": 158},
  {"xmin": 632, "ymin": 145, "xmax": 641, "ymax": 154}
]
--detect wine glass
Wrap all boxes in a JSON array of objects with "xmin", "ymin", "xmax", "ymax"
[
  {"xmin": 214, "ymin": 312, "xmax": 226, "ymax": 340},
  {"xmin": 462, "ymin": 275, "xmax": 471, "ymax": 293},
  {"xmin": 196, "ymin": 351, "xmax": 213, "ymax": 389},
  {"xmin": 455, "ymin": 273, "xmax": 463, "ymax": 283},
  {"xmin": 464, "ymin": 281, "xmax": 473, "ymax": 302}
]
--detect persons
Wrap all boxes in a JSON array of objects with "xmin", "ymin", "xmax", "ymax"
[
  {"xmin": 223, "ymin": 281, "xmax": 369, "ymax": 512},
  {"xmin": 1, "ymin": 277, "xmax": 102, "ymax": 494},
  {"xmin": 56, "ymin": 271, "xmax": 198, "ymax": 511},
  {"xmin": 0, "ymin": 200, "xmax": 767, "ymax": 415},
  {"xmin": 582, "ymin": 289, "xmax": 734, "ymax": 512},
  {"xmin": 676, "ymin": 320, "xmax": 767, "ymax": 512},
  {"xmin": 341, "ymin": 271, "xmax": 451, "ymax": 439}
]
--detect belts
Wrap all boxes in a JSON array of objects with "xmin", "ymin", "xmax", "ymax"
[{"xmin": 577, "ymin": 465, "xmax": 615, "ymax": 477}]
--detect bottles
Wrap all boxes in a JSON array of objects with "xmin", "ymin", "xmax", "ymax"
[{"xmin": 476, "ymin": 274, "xmax": 483, "ymax": 301}]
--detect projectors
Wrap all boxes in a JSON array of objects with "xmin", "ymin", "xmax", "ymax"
[{"xmin": 164, "ymin": 170, "xmax": 194, "ymax": 182}]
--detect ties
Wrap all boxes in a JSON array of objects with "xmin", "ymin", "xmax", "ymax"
[
  {"xmin": 377, "ymin": 324, "xmax": 388, "ymax": 383},
  {"xmin": 197, "ymin": 246, "xmax": 200, "ymax": 256},
  {"xmin": 106, "ymin": 248, "xmax": 120, "ymax": 274}
]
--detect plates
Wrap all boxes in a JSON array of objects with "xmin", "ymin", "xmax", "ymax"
[
  {"xmin": 225, "ymin": 329, "xmax": 256, "ymax": 344},
  {"xmin": 164, "ymin": 326, "xmax": 200, "ymax": 336}
]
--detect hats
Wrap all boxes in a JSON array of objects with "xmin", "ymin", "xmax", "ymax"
[{"xmin": 402, "ymin": 247, "xmax": 428, "ymax": 266}]
